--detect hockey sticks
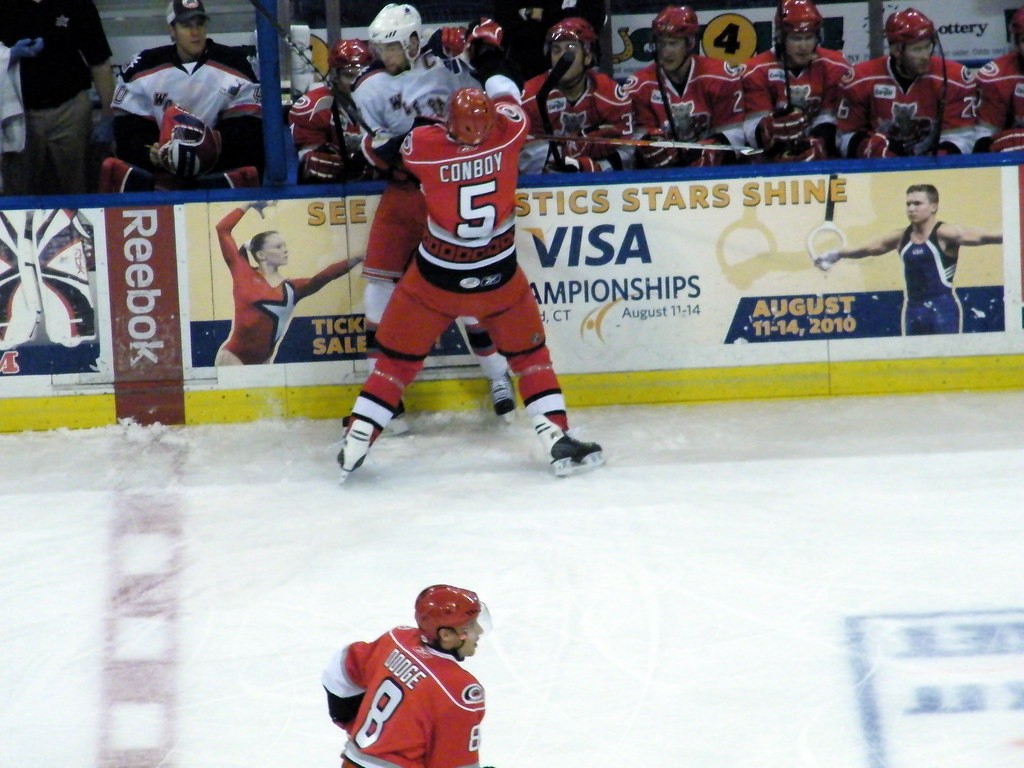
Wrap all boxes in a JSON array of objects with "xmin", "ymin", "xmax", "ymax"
[
  {"xmin": 774, "ymin": 0, "xmax": 793, "ymax": 111},
  {"xmin": 247, "ymin": 0, "xmax": 373, "ymax": 137},
  {"xmin": 329, "ymin": 95, "xmax": 346, "ymax": 156},
  {"xmin": 527, "ymin": 132, "xmax": 763, "ymax": 159},
  {"xmin": 927, "ymin": 24, "xmax": 952, "ymax": 156},
  {"xmin": 650, "ymin": 19, "xmax": 680, "ymax": 141},
  {"xmin": 535, "ymin": 49, "xmax": 577, "ymax": 159}
]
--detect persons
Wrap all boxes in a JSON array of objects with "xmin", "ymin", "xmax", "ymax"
[
  {"xmin": 333, "ymin": 36, "xmax": 602, "ymax": 472},
  {"xmin": 738, "ymin": 0, "xmax": 855, "ymax": 166},
  {"xmin": 287, "ymin": 3, "xmax": 502, "ymax": 185},
  {"xmin": 214, "ymin": 200, "xmax": 367, "ymax": 365},
  {"xmin": 322, "ymin": 585, "xmax": 493, "ymax": 768},
  {"xmin": 523, "ymin": 18, "xmax": 632, "ymax": 173},
  {"xmin": 834, "ymin": 8, "xmax": 978, "ymax": 161},
  {"xmin": 813, "ymin": 185, "xmax": 1002, "ymax": 334},
  {"xmin": 977, "ymin": 5, "xmax": 1024, "ymax": 153},
  {"xmin": 624, "ymin": 7, "xmax": 743, "ymax": 168},
  {"xmin": 0, "ymin": 0, "xmax": 264, "ymax": 197}
]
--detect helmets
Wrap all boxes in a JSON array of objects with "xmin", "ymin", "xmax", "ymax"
[
  {"xmin": 546, "ymin": 17, "xmax": 598, "ymax": 44},
  {"xmin": 652, "ymin": 5, "xmax": 699, "ymax": 36},
  {"xmin": 327, "ymin": 39, "xmax": 369, "ymax": 68},
  {"xmin": 415, "ymin": 585, "xmax": 481, "ymax": 644},
  {"xmin": 448, "ymin": 87, "xmax": 496, "ymax": 146},
  {"xmin": 157, "ymin": 107, "xmax": 219, "ymax": 180},
  {"xmin": 886, "ymin": 7, "xmax": 935, "ymax": 44},
  {"xmin": 1012, "ymin": 8, "xmax": 1024, "ymax": 38},
  {"xmin": 367, "ymin": 3, "xmax": 423, "ymax": 68},
  {"xmin": 775, "ymin": 0, "xmax": 823, "ymax": 33}
]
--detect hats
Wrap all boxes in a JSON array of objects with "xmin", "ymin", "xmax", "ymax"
[{"xmin": 166, "ymin": 0, "xmax": 211, "ymax": 26}]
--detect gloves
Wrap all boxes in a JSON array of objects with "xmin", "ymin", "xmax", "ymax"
[
  {"xmin": 634, "ymin": 133, "xmax": 678, "ymax": 168},
  {"xmin": 857, "ymin": 131, "xmax": 896, "ymax": 157},
  {"xmin": 554, "ymin": 128, "xmax": 631, "ymax": 174},
  {"xmin": 431, "ymin": 25, "xmax": 468, "ymax": 60},
  {"xmin": 301, "ymin": 149, "xmax": 342, "ymax": 184},
  {"xmin": 760, "ymin": 107, "xmax": 826, "ymax": 161},
  {"xmin": 689, "ymin": 132, "xmax": 735, "ymax": 166},
  {"xmin": 89, "ymin": 114, "xmax": 114, "ymax": 145},
  {"xmin": 464, "ymin": 16, "xmax": 504, "ymax": 47},
  {"xmin": 6, "ymin": 37, "xmax": 43, "ymax": 72},
  {"xmin": 371, "ymin": 129, "xmax": 402, "ymax": 164},
  {"xmin": 990, "ymin": 129, "xmax": 1024, "ymax": 152}
]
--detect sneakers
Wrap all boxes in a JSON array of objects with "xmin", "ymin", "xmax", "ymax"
[
  {"xmin": 487, "ymin": 373, "xmax": 518, "ymax": 424},
  {"xmin": 341, "ymin": 397, "xmax": 410, "ymax": 438},
  {"xmin": 336, "ymin": 419, "xmax": 375, "ymax": 483},
  {"xmin": 547, "ymin": 427, "xmax": 607, "ymax": 476}
]
